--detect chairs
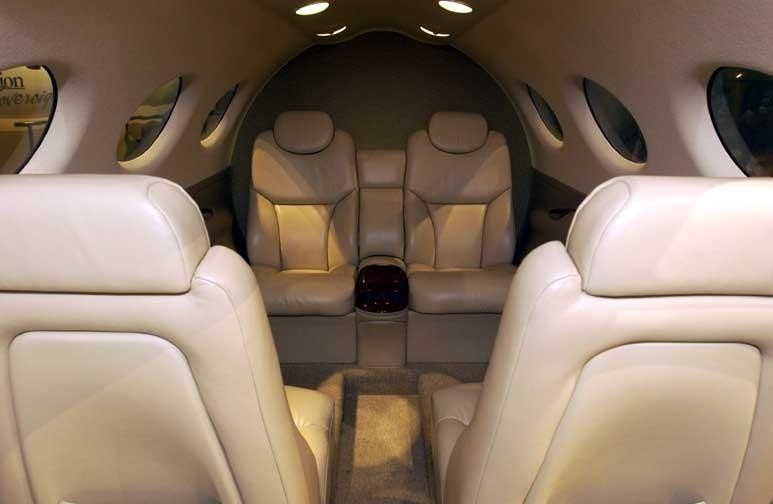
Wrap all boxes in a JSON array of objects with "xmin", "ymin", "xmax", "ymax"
[
  {"xmin": 245, "ymin": 110, "xmax": 358, "ymax": 367},
  {"xmin": 431, "ymin": 174, "xmax": 773, "ymax": 504},
  {"xmin": 1, "ymin": 171, "xmax": 337, "ymax": 504},
  {"xmin": 403, "ymin": 108, "xmax": 518, "ymax": 364}
]
